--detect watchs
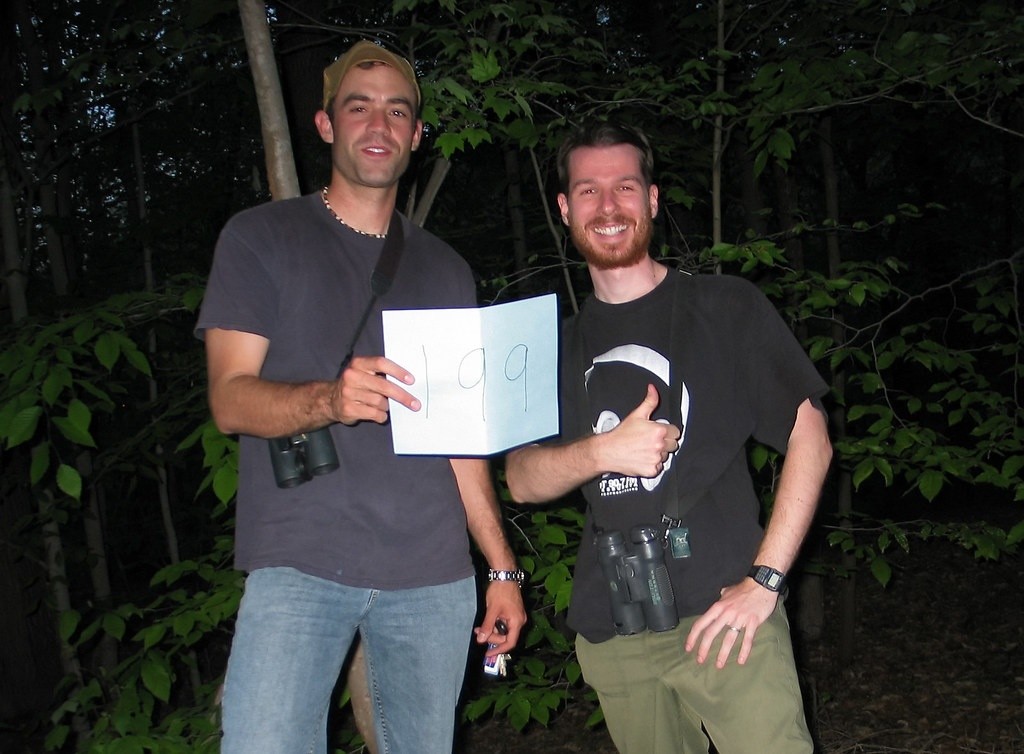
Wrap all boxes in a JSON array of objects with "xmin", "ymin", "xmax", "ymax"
[{"xmin": 748, "ymin": 565, "xmax": 787, "ymax": 593}]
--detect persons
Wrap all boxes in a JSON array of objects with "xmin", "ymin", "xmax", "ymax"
[
  {"xmin": 197, "ymin": 32, "xmax": 527, "ymax": 754},
  {"xmin": 503, "ymin": 121, "xmax": 835, "ymax": 754}
]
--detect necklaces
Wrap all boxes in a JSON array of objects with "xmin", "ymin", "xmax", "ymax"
[{"xmin": 323, "ymin": 187, "xmax": 388, "ymax": 240}]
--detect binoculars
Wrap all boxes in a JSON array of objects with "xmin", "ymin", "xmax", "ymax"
[
  {"xmin": 267, "ymin": 426, "xmax": 339, "ymax": 489},
  {"xmin": 596, "ymin": 523, "xmax": 680, "ymax": 636}
]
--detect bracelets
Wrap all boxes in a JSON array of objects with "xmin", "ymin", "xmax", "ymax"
[{"xmin": 488, "ymin": 569, "xmax": 524, "ymax": 582}]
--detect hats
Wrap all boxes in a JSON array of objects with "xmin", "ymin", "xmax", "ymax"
[{"xmin": 323, "ymin": 40, "xmax": 421, "ymax": 115}]
